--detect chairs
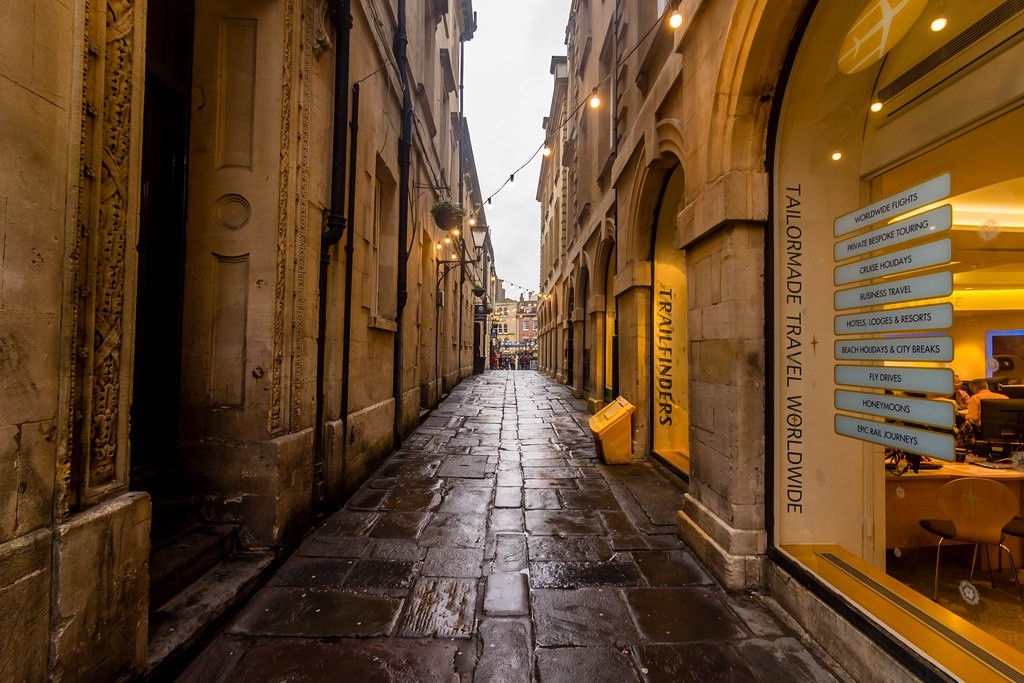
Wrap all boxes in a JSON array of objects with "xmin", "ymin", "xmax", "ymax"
[
  {"xmin": 998, "ymin": 516, "xmax": 1024, "ymax": 573},
  {"xmin": 911, "ymin": 477, "xmax": 1024, "ymax": 605},
  {"xmin": 965, "ymin": 399, "xmax": 1024, "ymax": 463}
]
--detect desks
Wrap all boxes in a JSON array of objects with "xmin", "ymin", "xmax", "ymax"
[{"xmin": 885, "ymin": 452, "xmax": 1024, "ymax": 571}]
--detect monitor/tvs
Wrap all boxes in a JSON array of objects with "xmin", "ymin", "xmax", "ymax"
[{"xmin": 980, "ymin": 399, "xmax": 1024, "ymax": 442}]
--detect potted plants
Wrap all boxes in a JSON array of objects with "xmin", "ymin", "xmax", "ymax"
[
  {"xmin": 472, "ymin": 284, "xmax": 485, "ymax": 297},
  {"xmin": 429, "ymin": 200, "xmax": 466, "ymax": 231}
]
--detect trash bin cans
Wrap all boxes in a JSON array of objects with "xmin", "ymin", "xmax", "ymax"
[{"xmin": 588, "ymin": 395, "xmax": 636, "ymax": 465}]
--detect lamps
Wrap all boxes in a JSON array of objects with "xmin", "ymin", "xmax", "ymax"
[
  {"xmin": 542, "ymin": 140, "xmax": 551, "ymax": 157},
  {"xmin": 451, "ymin": 252, "xmax": 456, "ymax": 259},
  {"xmin": 487, "ymin": 274, "xmax": 552, "ymax": 352},
  {"xmin": 437, "ymin": 241, "xmax": 441, "ymax": 249},
  {"xmin": 454, "ymin": 227, "xmax": 459, "ymax": 235},
  {"xmin": 435, "ymin": 215, "xmax": 490, "ymax": 288},
  {"xmin": 590, "ymin": 87, "xmax": 600, "ymax": 108},
  {"xmin": 670, "ymin": 0, "xmax": 683, "ymax": 28},
  {"xmin": 470, "ymin": 215, "xmax": 475, "ymax": 224},
  {"xmin": 445, "ymin": 235, "xmax": 450, "ymax": 243}
]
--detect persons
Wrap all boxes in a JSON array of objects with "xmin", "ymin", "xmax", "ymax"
[
  {"xmin": 933, "ymin": 375, "xmax": 1010, "ymax": 436},
  {"xmin": 499, "ymin": 354, "xmax": 530, "ymax": 370}
]
